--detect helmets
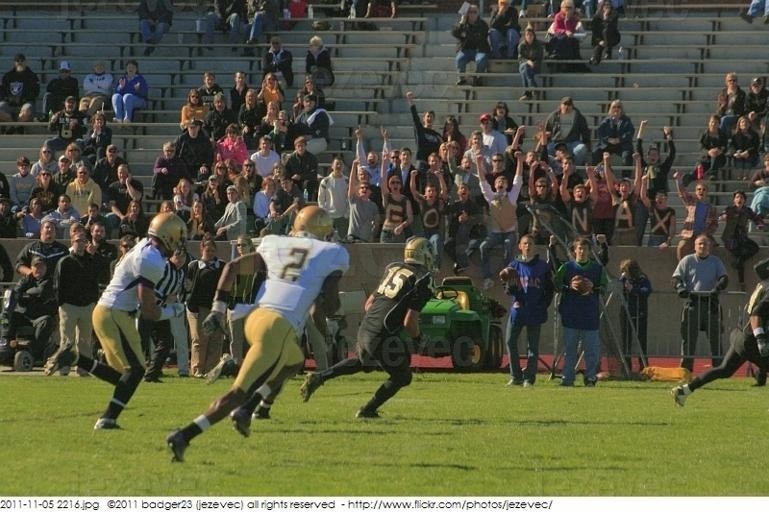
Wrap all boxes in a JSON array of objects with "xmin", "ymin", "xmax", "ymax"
[
  {"xmin": 291, "ymin": 206, "xmax": 335, "ymax": 242},
  {"xmin": 404, "ymin": 238, "xmax": 438, "ymax": 273},
  {"xmin": 145, "ymin": 212, "xmax": 189, "ymax": 255}
]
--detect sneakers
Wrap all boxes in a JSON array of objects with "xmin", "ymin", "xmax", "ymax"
[
  {"xmin": 299, "ymin": 372, "xmax": 324, "ymax": 402},
  {"xmin": 253, "ymin": 407, "xmax": 270, "ymax": 419},
  {"xmin": 669, "ymin": 386, "xmax": 686, "ymax": 405},
  {"xmin": 94, "ymin": 419, "xmax": 121, "ymax": 429},
  {"xmin": 42, "ymin": 337, "xmax": 76, "ymax": 378},
  {"xmin": 354, "ymin": 406, "xmax": 380, "ymax": 419},
  {"xmin": 230, "ymin": 406, "xmax": 251, "ymax": 438},
  {"xmin": 166, "ymin": 430, "xmax": 188, "ymax": 462},
  {"xmin": 204, "ymin": 353, "xmax": 234, "ymax": 386}
]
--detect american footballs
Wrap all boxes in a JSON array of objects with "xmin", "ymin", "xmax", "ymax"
[
  {"xmin": 500, "ymin": 267, "xmax": 518, "ymax": 281},
  {"xmin": 572, "ymin": 275, "xmax": 591, "ymax": 295}
]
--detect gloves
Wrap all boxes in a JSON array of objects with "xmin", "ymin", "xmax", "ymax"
[
  {"xmin": 201, "ymin": 313, "xmax": 229, "ymax": 338},
  {"xmin": 756, "ymin": 334, "xmax": 769, "ymax": 358}
]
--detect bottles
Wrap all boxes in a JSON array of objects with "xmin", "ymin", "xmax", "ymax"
[
  {"xmin": 303, "ymin": 188, "xmax": 309, "ymax": 204},
  {"xmin": 48, "ymin": 109, "xmax": 54, "ymax": 123},
  {"xmin": 618, "ymin": 46, "xmax": 625, "ymax": 60},
  {"xmin": 341, "ymin": 137, "xmax": 347, "ymax": 150},
  {"xmin": 697, "ymin": 164, "xmax": 704, "ymax": 180}
]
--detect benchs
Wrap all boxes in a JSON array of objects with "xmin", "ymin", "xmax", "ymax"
[{"xmin": 2, "ymin": 2, "xmax": 767, "ymax": 238}]
